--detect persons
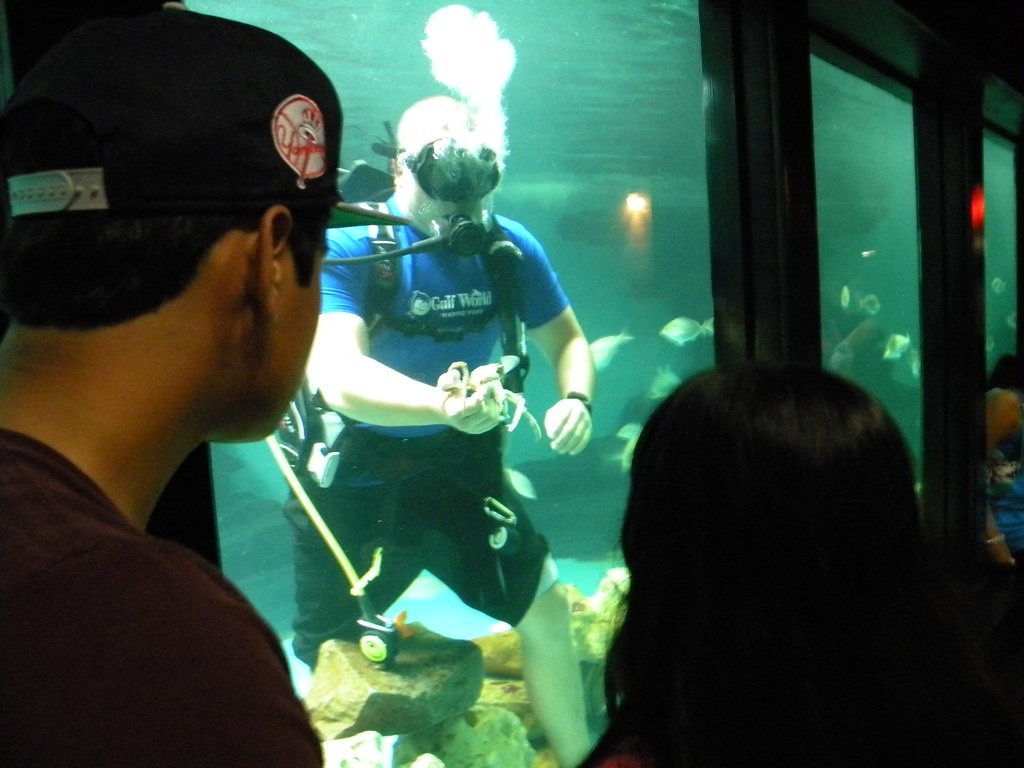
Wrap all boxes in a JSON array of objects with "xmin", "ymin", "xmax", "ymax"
[
  {"xmin": 281, "ymin": 94, "xmax": 595, "ymax": 767},
  {"xmin": 0, "ymin": 0, "xmax": 344, "ymax": 768},
  {"xmin": 574, "ymin": 351, "xmax": 1024, "ymax": 768}
]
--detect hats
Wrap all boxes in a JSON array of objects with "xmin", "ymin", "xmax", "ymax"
[{"xmin": 1, "ymin": 6, "xmax": 404, "ymax": 227}]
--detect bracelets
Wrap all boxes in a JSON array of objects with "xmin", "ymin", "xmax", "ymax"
[
  {"xmin": 984, "ymin": 533, "xmax": 1005, "ymax": 546},
  {"xmin": 566, "ymin": 391, "xmax": 593, "ymax": 415}
]
